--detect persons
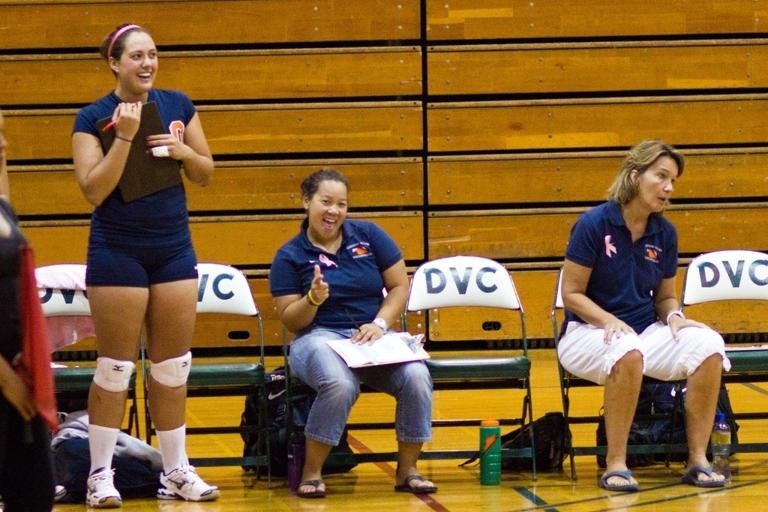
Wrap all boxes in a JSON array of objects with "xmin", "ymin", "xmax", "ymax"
[
  {"xmin": 0, "ymin": 113, "xmax": 59, "ymax": 512},
  {"xmin": 558, "ymin": 138, "xmax": 727, "ymax": 492},
  {"xmin": 270, "ymin": 169, "xmax": 438, "ymax": 498},
  {"xmin": 72, "ymin": 23, "xmax": 219, "ymax": 508}
]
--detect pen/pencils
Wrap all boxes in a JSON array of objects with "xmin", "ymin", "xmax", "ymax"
[
  {"xmin": 101, "ymin": 121, "xmax": 116, "ymax": 132},
  {"xmin": 351, "ymin": 319, "xmax": 361, "ymax": 333}
]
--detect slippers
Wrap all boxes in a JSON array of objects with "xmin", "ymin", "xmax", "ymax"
[
  {"xmin": 680, "ymin": 464, "xmax": 725, "ymax": 486},
  {"xmin": 599, "ymin": 468, "xmax": 641, "ymax": 490},
  {"xmin": 297, "ymin": 478, "xmax": 326, "ymax": 499},
  {"xmin": 393, "ymin": 474, "xmax": 438, "ymax": 494}
]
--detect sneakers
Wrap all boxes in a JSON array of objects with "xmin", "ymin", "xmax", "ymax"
[
  {"xmin": 158, "ymin": 466, "xmax": 219, "ymax": 502},
  {"xmin": 85, "ymin": 468, "xmax": 122, "ymax": 509}
]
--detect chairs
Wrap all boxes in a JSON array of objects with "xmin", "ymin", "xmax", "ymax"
[
  {"xmin": 403, "ymin": 255, "xmax": 540, "ymax": 485},
  {"xmin": 551, "ymin": 266, "xmax": 686, "ymax": 477},
  {"xmin": 34, "ymin": 264, "xmax": 142, "ymax": 439},
  {"xmin": 680, "ymin": 250, "xmax": 768, "ymax": 468},
  {"xmin": 141, "ymin": 260, "xmax": 273, "ymax": 488},
  {"xmin": 279, "ymin": 287, "xmax": 405, "ymax": 489}
]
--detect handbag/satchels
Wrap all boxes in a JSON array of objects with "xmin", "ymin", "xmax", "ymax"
[
  {"xmin": 500, "ymin": 412, "xmax": 572, "ymax": 470},
  {"xmin": 239, "ymin": 362, "xmax": 357, "ymax": 476},
  {"xmin": 594, "ymin": 380, "xmax": 739, "ymax": 468}
]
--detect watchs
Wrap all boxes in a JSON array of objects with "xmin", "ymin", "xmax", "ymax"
[{"xmin": 372, "ymin": 318, "xmax": 387, "ymax": 335}]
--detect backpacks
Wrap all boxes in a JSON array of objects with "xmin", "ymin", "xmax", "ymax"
[{"xmin": 48, "ymin": 413, "xmax": 168, "ymax": 504}]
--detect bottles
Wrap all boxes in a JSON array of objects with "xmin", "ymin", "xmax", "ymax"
[
  {"xmin": 478, "ymin": 414, "xmax": 501, "ymax": 486},
  {"xmin": 286, "ymin": 440, "xmax": 302, "ymax": 492},
  {"xmin": 709, "ymin": 413, "xmax": 732, "ymax": 485}
]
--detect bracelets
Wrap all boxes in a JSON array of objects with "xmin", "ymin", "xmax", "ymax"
[
  {"xmin": 115, "ymin": 135, "xmax": 132, "ymax": 143},
  {"xmin": 667, "ymin": 311, "xmax": 685, "ymax": 327},
  {"xmin": 307, "ymin": 290, "xmax": 322, "ymax": 306}
]
